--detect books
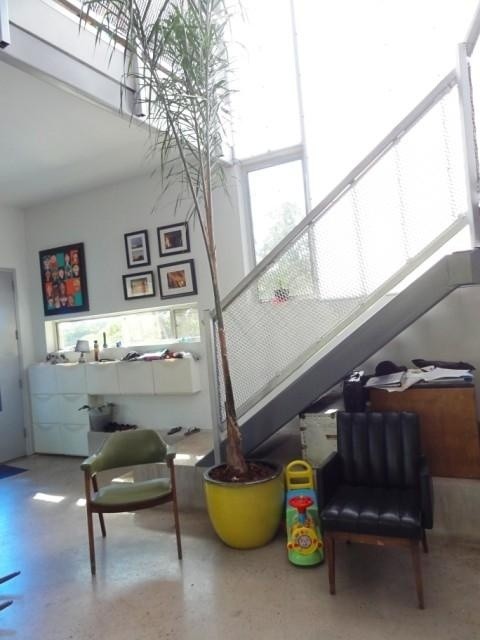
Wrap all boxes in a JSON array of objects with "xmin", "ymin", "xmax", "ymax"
[{"xmin": 363, "ymin": 370, "xmax": 406, "ymax": 388}]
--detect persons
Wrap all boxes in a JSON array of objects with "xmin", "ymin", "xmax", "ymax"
[{"xmin": 42, "ymin": 250, "xmax": 81, "ymax": 310}]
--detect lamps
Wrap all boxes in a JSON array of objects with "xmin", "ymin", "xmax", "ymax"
[{"xmin": 74, "ymin": 339, "xmax": 90, "ymax": 363}]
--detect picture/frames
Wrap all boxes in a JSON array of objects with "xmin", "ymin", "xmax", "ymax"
[
  {"xmin": 38, "ymin": 241, "xmax": 90, "ymax": 317},
  {"xmin": 120, "ymin": 221, "xmax": 198, "ymax": 301}
]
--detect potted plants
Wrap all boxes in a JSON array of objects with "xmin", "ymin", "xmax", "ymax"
[{"xmin": 72, "ymin": 0, "xmax": 285, "ymax": 550}]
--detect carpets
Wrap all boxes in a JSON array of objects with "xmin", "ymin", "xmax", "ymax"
[{"xmin": 0, "ymin": 463, "xmax": 29, "ymax": 480}]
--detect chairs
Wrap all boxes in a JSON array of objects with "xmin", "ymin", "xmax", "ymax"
[
  {"xmin": 80, "ymin": 429, "xmax": 183, "ymax": 575},
  {"xmin": 316, "ymin": 407, "xmax": 435, "ymax": 610}
]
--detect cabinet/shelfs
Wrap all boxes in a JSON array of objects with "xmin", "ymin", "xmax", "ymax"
[{"xmin": 27, "ymin": 356, "xmax": 199, "ymax": 459}]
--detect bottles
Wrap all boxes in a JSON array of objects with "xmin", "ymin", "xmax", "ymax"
[{"xmin": 94, "ymin": 341, "xmax": 99, "ymax": 360}]
